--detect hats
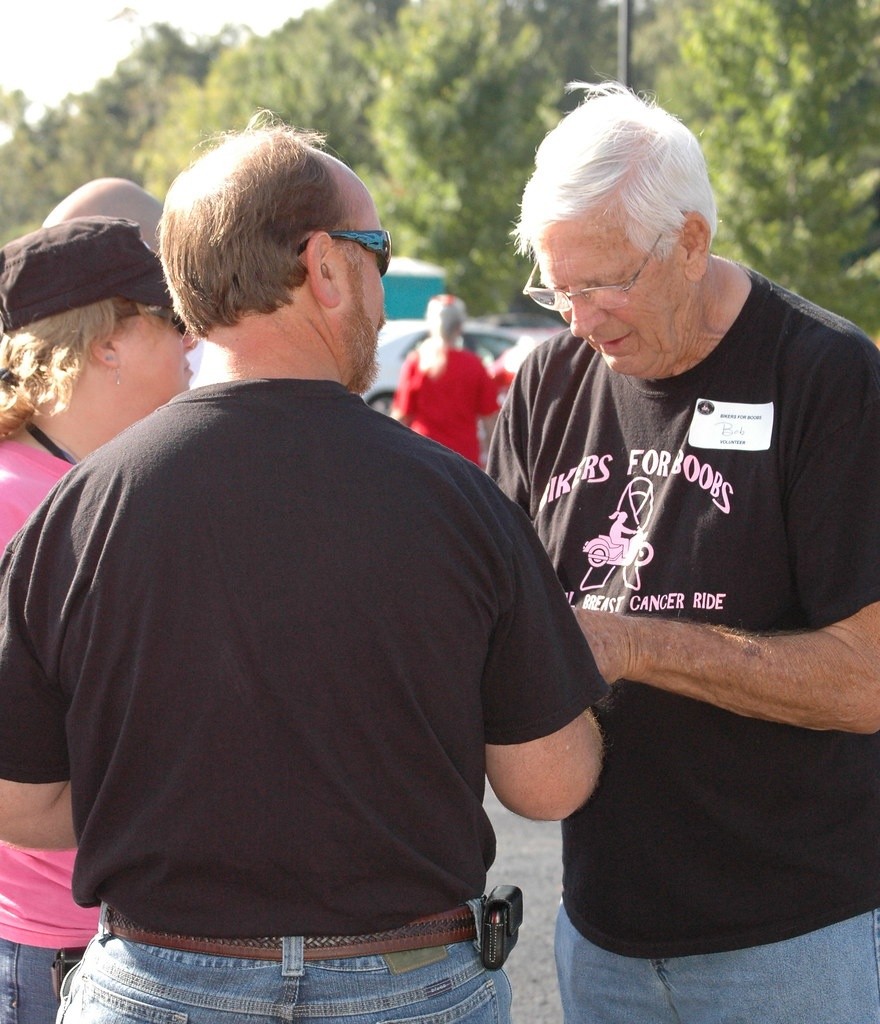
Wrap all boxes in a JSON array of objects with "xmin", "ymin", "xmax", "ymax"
[{"xmin": 0, "ymin": 216, "xmax": 180, "ymax": 332}]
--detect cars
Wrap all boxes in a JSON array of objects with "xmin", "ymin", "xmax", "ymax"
[{"xmin": 355, "ymin": 323, "xmax": 539, "ymax": 419}]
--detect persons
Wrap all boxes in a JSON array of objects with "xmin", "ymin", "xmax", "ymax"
[
  {"xmin": 484, "ymin": 77, "xmax": 880, "ymax": 1024},
  {"xmin": 0, "ymin": 111, "xmax": 604, "ymax": 1024}
]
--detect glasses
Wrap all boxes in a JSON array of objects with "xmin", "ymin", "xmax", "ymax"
[
  {"xmin": 150, "ymin": 309, "xmax": 187, "ymax": 335},
  {"xmin": 298, "ymin": 229, "xmax": 391, "ymax": 278},
  {"xmin": 522, "ymin": 230, "xmax": 660, "ymax": 313}
]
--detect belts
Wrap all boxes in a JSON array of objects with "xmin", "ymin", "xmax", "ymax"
[{"xmin": 104, "ymin": 903, "xmax": 477, "ymax": 962}]
